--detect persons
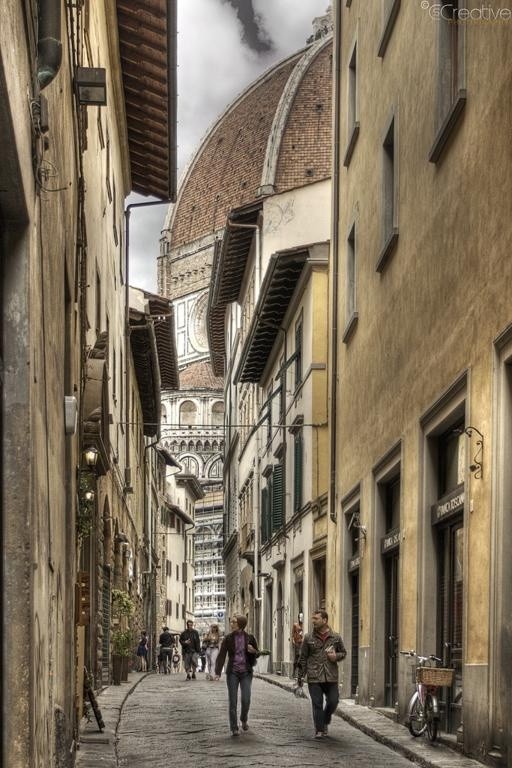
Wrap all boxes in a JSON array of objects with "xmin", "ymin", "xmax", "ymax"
[
  {"xmin": 137, "ymin": 629, "xmax": 147, "ymax": 671},
  {"xmin": 296, "ymin": 608, "xmax": 348, "ymax": 738},
  {"xmin": 198, "ymin": 632, "xmax": 207, "ymax": 671},
  {"xmin": 201, "ymin": 623, "xmax": 224, "ymax": 680},
  {"xmin": 159, "ymin": 626, "xmax": 178, "ymax": 673},
  {"xmin": 214, "ymin": 613, "xmax": 259, "ymax": 735},
  {"xmin": 292, "ymin": 622, "xmax": 304, "ymax": 691},
  {"xmin": 179, "ymin": 619, "xmax": 200, "ymax": 680}
]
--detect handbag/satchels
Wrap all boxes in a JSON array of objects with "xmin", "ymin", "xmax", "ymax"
[{"xmin": 247, "ymin": 652, "xmax": 257, "ymax": 666}]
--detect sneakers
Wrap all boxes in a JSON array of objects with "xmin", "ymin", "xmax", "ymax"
[
  {"xmin": 233, "ymin": 727, "xmax": 238, "ymax": 736},
  {"xmin": 315, "ymin": 725, "xmax": 328, "ymax": 738},
  {"xmin": 241, "ymin": 720, "xmax": 249, "ymax": 730}
]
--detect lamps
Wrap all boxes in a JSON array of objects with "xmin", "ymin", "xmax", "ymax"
[
  {"xmin": 76, "ymin": 487, "xmax": 95, "ymax": 507},
  {"xmin": 76, "ymin": 444, "xmax": 99, "ymax": 481}
]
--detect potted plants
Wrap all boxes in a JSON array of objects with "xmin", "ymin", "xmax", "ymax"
[{"xmin": 108, "ymin": 585, "xmax": 138, "ymax": 685}]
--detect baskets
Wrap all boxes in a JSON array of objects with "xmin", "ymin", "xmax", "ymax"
[{"xmin": 416, "ymin": 667, "xmax": 455, "ymax": 687}]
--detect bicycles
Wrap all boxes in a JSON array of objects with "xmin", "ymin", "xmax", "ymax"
[{"xmin": 400, "ymin": 651, "xmax": 455, "ymax": 742}]
